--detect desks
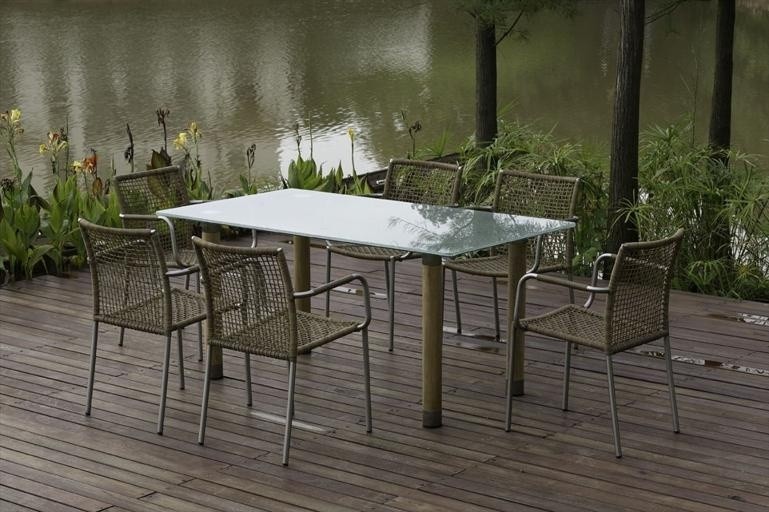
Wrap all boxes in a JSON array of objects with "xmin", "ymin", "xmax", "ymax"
[{"xmin": 156, "ymin": 188, "xmax": 576, "ymax": 428}]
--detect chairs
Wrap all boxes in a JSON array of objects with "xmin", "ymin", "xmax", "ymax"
[
  {"xmin": 325, "ymin": 159, "xmax": 462, "ymax": 353},
  {"xmin": 112, "ymin": 165, "xmax": 239, "ymax": 347},
  {"xmin": 441, "ymin": 169, "xmax": 581, "ymax": 350},
  {"xmin": 192, "ymin": 236, "xmax": 372, "ymax": 466},
  {"xmin": 504, "ymin": 227, "xmax": 687, "ymax": 459},
  {"xmin": 77, "ymin": 217, "xmax": 241, "ymax": 435}
]
